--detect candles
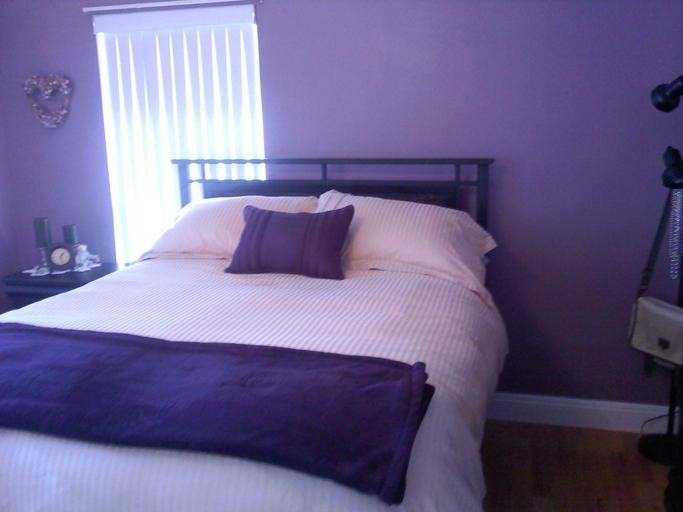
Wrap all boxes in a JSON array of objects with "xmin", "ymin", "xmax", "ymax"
[
  {"xmin": 63, "ymin": 225, "xmax": 77, "ymax": 245},
  {"xmin": 34, "ymin": 218, "xmax": 50, "ymax": 248}
]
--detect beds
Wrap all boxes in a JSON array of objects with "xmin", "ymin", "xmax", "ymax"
[{"xmin": 0, "ymin": 157, "xmax": 509, "ymax": 512}]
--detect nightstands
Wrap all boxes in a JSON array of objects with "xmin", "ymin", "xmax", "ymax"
[{"xmin": 4, "ymin": 262, "xmax": 115, "ymax": 309}]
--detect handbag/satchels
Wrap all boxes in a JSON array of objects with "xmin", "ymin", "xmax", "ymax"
[{"xmin": 631, "ymin": 295, "xmax": 683, "ymax": 365}]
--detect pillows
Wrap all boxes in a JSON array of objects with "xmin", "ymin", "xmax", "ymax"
[
  {"xmin": 139, "ymin": 195, "xmax": 317, "ymax": 260},
  {"xmin": 225, "ymin": 204, "xmax": 354, "ymax": 280},
  {"xmin": 315, "ymin": 188, "xmax": 499, "ymax": 308}
]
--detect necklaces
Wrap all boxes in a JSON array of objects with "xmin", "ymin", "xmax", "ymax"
[{"xmin": 666, "ymin": 187, "xmax": 682, "ymax": 283}]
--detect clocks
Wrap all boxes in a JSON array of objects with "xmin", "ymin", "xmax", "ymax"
[{"xmin": 46, "ymin": 243, "xmax": 74, "ymax": 269}]
